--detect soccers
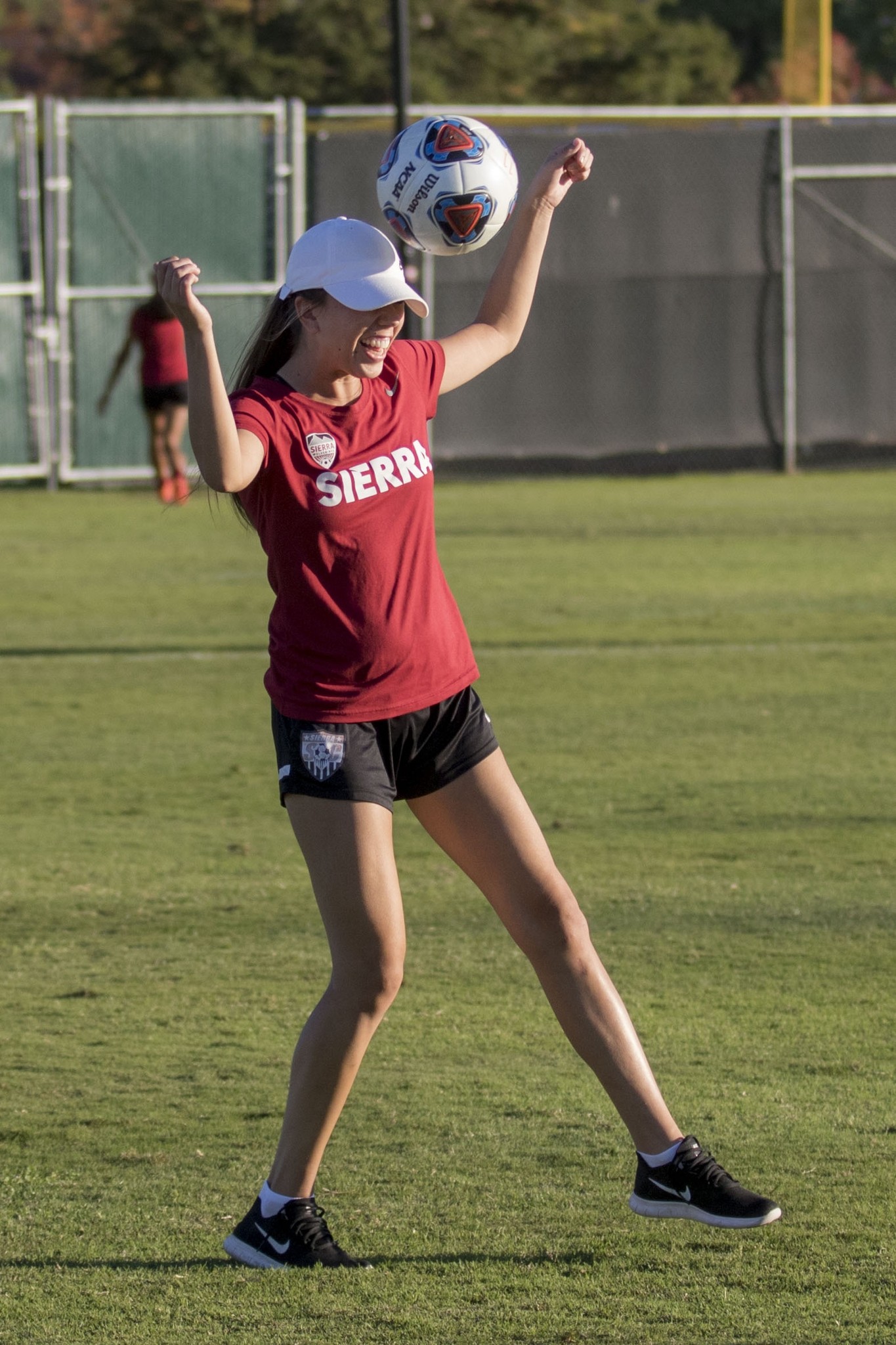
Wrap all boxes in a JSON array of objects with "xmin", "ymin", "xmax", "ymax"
[{"xmin": 375, "ymin": 112, "xmax": 520, "ymax": 259}]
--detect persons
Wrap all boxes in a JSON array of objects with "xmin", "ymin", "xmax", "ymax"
[
  {"xmin": 151, "ymin": 137, "xmax": 781, "ymax": 1274},
  {"xmin": 98, "ymin": 258, "xmax": 190, "ymax": 506}
]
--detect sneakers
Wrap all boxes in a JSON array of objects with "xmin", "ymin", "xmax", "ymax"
[
  {"xmin": 629, "ymin": 1133, "xmax": 783, "ymax": 1228},
  {"xmin": 223, "ymin": 1196, "xmax": 373, "ymax": 1272}
]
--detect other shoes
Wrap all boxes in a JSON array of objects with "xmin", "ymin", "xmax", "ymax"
[
  {"xmin": 174, "ymin": 472, "xmax": 187, "ymax": 502},
  {"xmin": 159, "ymin": 477, "xmax": 175, "ymax": 504}
]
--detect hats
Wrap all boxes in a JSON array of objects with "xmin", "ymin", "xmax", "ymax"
[{"xmin": 279, "ymin": 215, "xmax": 431, "ymax": 321}]
